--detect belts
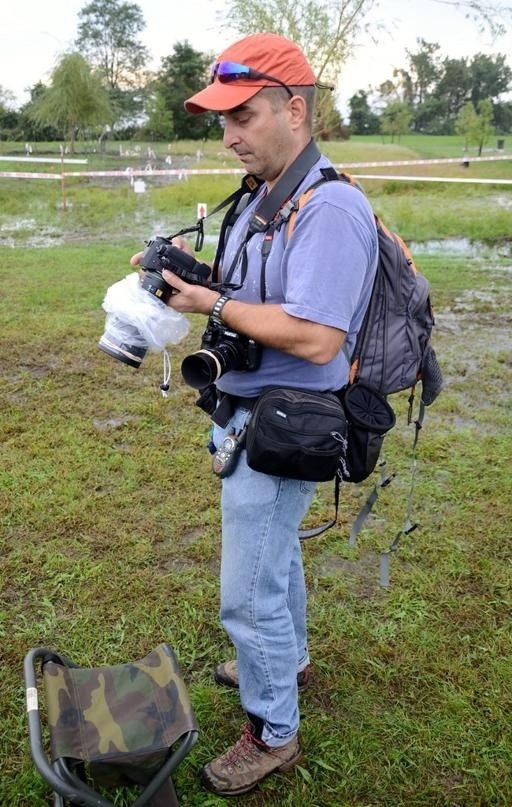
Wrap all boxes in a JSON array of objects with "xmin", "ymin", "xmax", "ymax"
[{"xmin": 208, "ymin": 386, "xmax": 260, "ymax": 427}]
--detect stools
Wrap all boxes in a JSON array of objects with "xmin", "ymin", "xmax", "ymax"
[{"xmin": 23, "ymin": 643, "xmax": 200, "ymax": 806}]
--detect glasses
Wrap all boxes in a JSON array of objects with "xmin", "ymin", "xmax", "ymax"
[{"xmin": 210, "ymin": 61, "xmax": 294, "ymax": 99}]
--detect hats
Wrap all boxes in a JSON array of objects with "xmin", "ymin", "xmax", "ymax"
[{"xmin": 183, "ymin": 32, "xmax": 318, "ymax": 111}]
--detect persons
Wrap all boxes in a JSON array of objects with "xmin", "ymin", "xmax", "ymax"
[{"xmin": 129, "ymin": 31, "xmax": 382, "ymax": 798}]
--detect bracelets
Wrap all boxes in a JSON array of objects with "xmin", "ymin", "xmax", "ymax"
[{"xmin": 209, "ymin": 294, "xmax": 232, "ymax": 331}]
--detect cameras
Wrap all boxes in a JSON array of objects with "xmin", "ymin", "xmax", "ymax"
[
  {"xmin": 98, "ymin": 236, "xmax": 213, "ymax": 369},
  {"xmin": 181, "ymin": 318, "xmax": 263, "ymax": 390}
]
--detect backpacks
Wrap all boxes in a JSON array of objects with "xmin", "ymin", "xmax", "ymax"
[{"xmin": 281, "ymin": 170, "xmax": 444, "ymax": 409}]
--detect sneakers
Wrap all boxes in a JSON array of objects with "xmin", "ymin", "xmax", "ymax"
[
  {"xmin": 215, "ymin": 658, "xmax": 311, "ymax": 693},
  {"xmin": 198, "ymin": 723, "xmax": 305, "ymax": 797}
]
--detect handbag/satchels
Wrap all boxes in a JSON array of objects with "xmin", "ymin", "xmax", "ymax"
[{"xmin": 244, "ymin": 385, "xmax": 343, "ymax": 483}]
